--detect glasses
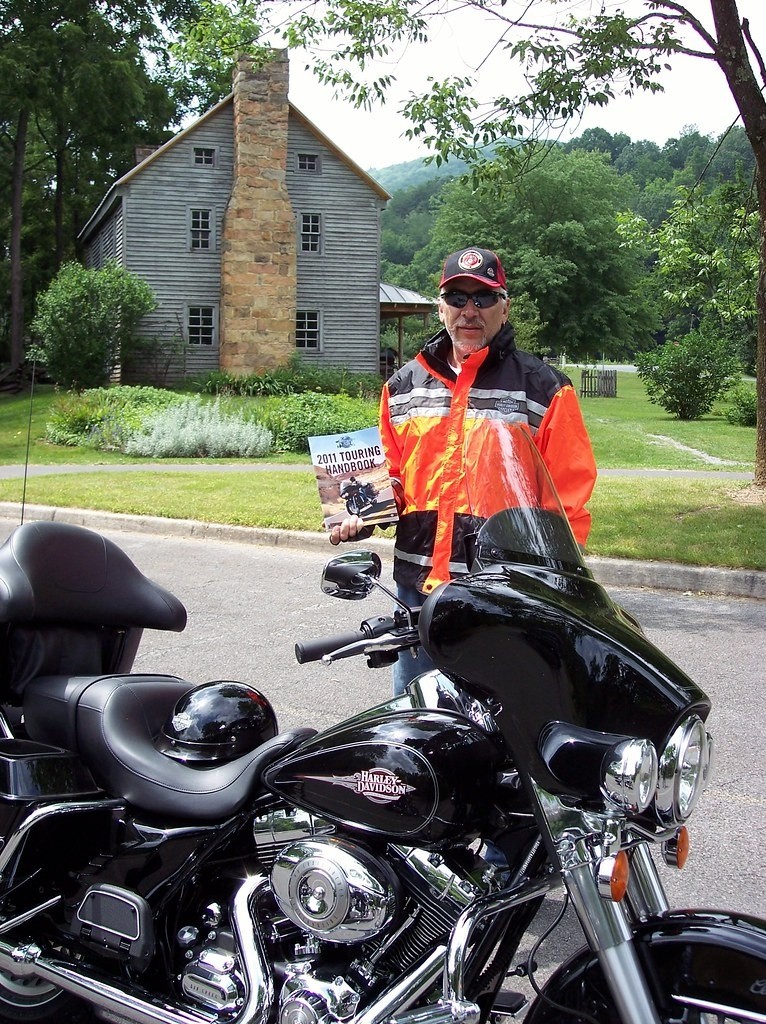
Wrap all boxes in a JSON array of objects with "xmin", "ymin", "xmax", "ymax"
[{"xmin": 440, "ymin": 287, "xmax": 506, "ymax": 308}]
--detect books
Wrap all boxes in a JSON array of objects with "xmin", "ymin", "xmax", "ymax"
[{"xmin": 308, "ymin": 425, "xmax": 399, "ymax": 532}]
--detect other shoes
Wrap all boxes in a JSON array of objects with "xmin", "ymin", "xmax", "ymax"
[{"xmin": 365, "ymin": 501, "xmax": 370, "ymax": 505}]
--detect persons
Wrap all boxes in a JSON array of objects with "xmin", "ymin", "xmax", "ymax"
[
  {"xmin": 320, "ymin": 248, "xmax": 598, "ymax": 899},
  {"xmin": 349, "ymin": 475, "xmax": 361, "ymax": 485}
]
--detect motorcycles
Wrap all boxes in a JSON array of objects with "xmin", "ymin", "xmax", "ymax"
[{"xmin": 0, "ymin": 417, "xmax": 766, "ymax": 1024}]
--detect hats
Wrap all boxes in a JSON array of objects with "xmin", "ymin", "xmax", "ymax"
[
  {"xmin": 350, "ymin": 476, "xmax": 355, "ymax": 482},
  {"xmin": 439, "ymin": 247, "xmax": 507, "ymax": 289}
]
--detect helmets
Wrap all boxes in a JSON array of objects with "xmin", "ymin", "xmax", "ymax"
[{"xmin": 154, "ymin": 681, "xmax": 279, "ymax": 762}]
